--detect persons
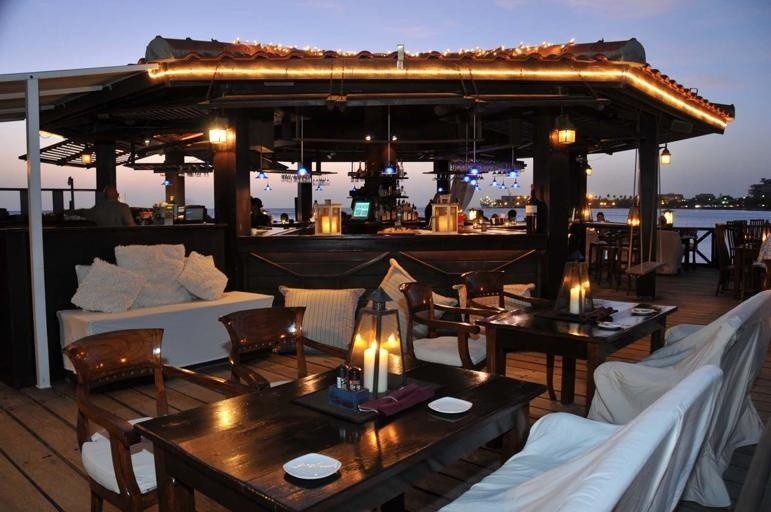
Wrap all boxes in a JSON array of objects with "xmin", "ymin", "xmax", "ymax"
[
  {"xmin": 597, "ymin": 212, "xmax": 605, "ymax": 222},
  {"xmin": 91, "ymin": 183, "xmax": 136, "ymax": 226},
  {"xmin": 251, "ymin": 197, "xmax": 272, "ymax": 226},
  {"xmin": 424, "ymin": 192, "xmax": 517, "ymax": 232},
  {"xmin": 281, "ymin": 213, "xmax": 288, "ymax": 222}
]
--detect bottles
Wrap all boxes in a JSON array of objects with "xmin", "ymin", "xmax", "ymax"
[
  {"xmin": 168, "ymin": 195, "xmax": 177, "ymax": 222},
  {"xmin": 312, "ymin": 200, "xmax": 318, "ymax": 215},
  {"xmin": 373, "ymin": 200, "xmax": 417, "ymax": 222},
  {"xmin": 335, "ymin": 364, "xmax": 360, "ymax": 393}
]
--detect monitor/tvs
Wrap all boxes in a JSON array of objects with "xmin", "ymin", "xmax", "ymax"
[
  {"xmin": 183, "ymin": 205, "xmax": 207, "ymax": 223},
  {"xmin": 350, "ymin": 202, "xmax": 370, "ymax": 220}
]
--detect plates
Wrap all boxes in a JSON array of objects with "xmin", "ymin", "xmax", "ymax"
[
  {"xmin": 631, "ymin": 307, "xmax": 655, "ymax": 315},
  {"xmin": 426, "ymin": 396, "xmax": 474, "ymax": 414},
  {"xmin": 598, "ymin": 321, "xmax": 623, "ymax": 329},
  {"xmin": 281, "ymin": 453, "xmax": 341, "ymax": 480},
  {"xmin": 597, "ymin": 328, "xmax": 622, "ymax": 332}
]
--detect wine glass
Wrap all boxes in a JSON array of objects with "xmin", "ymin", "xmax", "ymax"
[
  {"xmin": 279, "ymin": 173, "xmax": 330, "ymax": 186},
  {"xmin": 177, "ymin": 166, "xmax": 208, "ymax": 177}
]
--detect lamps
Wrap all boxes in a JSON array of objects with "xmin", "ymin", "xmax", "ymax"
[
  {"xmin": 161, "ymin": 178, "xmax": 174, "ymax": 186},
  {"xmin": 661, "ymin": 207, "xmax": 677, "ymax": 228},
  {"xmin": 432, "ymin": 193, "xmax": 460, "ymax": 234},
  {"xmin": 659, "ymin": 142, "xmax": 674, "ymax": 166},
  {"xmin": 254, "ymin": 105, "xmax": 522, "ymax": 192},
  {"xmin": 314, "ymin": 198, "xmax": 343, "ymax": 235},
  {"xmin": 586, "ymin": 162, "xmax": 594, "ymax": 176},
  {"xmin": 208, "ymin": 106, "xmax": 229, "ymax": 146},
  {"xmin": 549, "ymin": 104, "xmax": 578, "ymax": 146}
]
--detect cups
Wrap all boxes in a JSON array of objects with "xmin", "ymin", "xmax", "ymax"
[
  {"xmin": 472, "ymin": 217, "xmax": 485, "ymax": 232},
  {"xmin": 177, "ymin": 205, "xmax": 184, "ymax": 221},
  {"xmin": 288, "ymin": 218, "xmax": 293, "ymax": 223},
  {"xmin": 489, "ymin": 218, "xmax": 515, "ymax": 226},
  {"xmin": 281, "ymin": 216, "xmax": 285, "ymax": 223}
]
